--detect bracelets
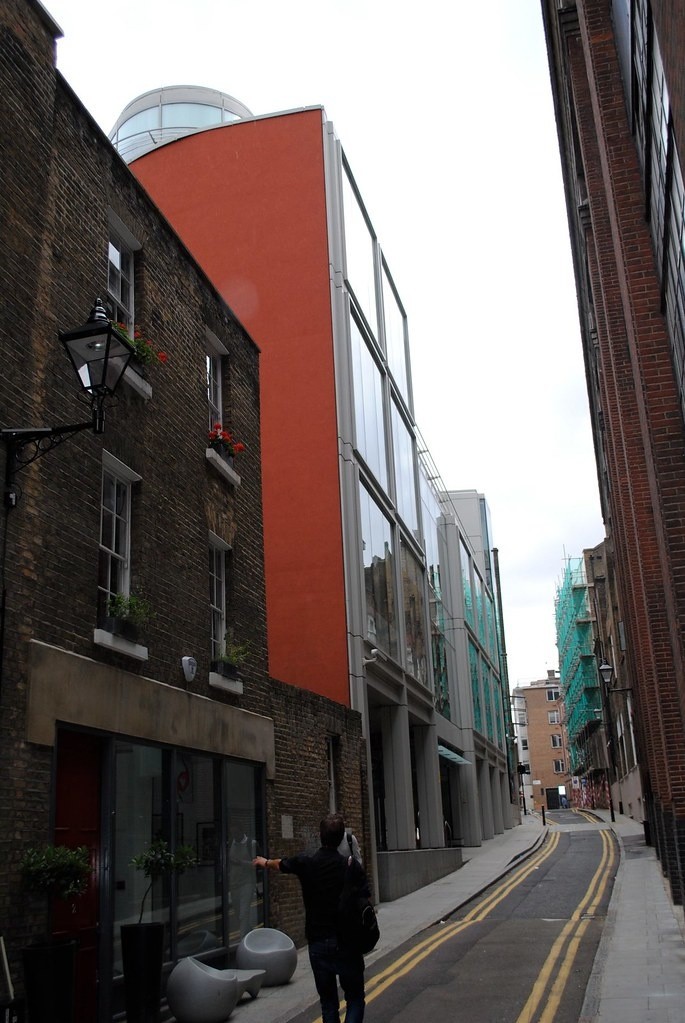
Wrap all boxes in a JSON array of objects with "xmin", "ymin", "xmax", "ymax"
[{"xmin": 265, "ymin": 860, "xmax": 268, "ymax": 867}]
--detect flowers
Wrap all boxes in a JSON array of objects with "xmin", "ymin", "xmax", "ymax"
[
  {"xmin": 109, "ymin": 318, "xmax": 166, "ymax": 375},
  {"xmin": 208, "ymin": 423, "xmax": 245, "ymax": 459}
]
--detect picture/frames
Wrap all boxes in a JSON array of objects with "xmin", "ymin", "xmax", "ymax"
[
  {"xmin": 196, "ymin": 821, "xmax": 215, "ymax": 867},
  {"xmin": 151, "ymin": 813, "xmax": 184, "ymax": 851}
]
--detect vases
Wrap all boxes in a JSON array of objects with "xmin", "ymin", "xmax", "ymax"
[
  {"xmin": 209, "ymin": 443, "xmax": 233, "ymax": 469},
  {"xmin": 129, "ymin": 356, "xmax": 147, "ymax": 380}
]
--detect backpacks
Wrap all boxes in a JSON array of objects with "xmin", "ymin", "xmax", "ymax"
[{"xmin": 336, "ymin": 856, "xmax": 379, "ymax": 958}]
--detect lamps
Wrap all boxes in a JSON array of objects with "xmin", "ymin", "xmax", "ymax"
[
  {"xmin": 599, "ymin": 657, "xmax": 635, "ymax": 699},
  {"xmin": 0, "ymin": 297, "xmax": 136, "ymax": 507}
]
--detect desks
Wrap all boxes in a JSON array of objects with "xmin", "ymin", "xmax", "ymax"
[{"xmin": 222, "ymin": 969, "xmax": 266, "ymax": 1004}]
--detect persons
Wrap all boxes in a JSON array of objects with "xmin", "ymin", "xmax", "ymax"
[
  {"xmin": 227, "ymin": 820, "xmax": 261, "ymax": 941},
  {"xmin": 337, "ymin": 832, "xmax": 362, "ymax": 866},
  {"xmin": 562, "ymin": 796, "xmax": 568, "ymax": 809},
  {"xmin": 253, "ymin": 815, "xmax": 372, "ymax": 1023}
]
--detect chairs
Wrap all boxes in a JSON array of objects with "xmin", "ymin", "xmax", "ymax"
[
  {"xmin": 166, "ymin": 930, "xmax": 221, "ymax": 960},
  {"xmin": 235, "ymin": 926, "xmax": 297, "ymax": 987},
  {"xmin": 166, "ymin": 956, "xmax": 240, "ymax": 1023}
]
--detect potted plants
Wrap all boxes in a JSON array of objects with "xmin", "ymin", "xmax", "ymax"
[
  {"xmin": 121, "ymin": 840, "xmax": 200, "ymax": 1023},
  {"xmin": 98, "ymin": 593, "xmax": 157, "ymax": 643},
  {"xmin": 16, "ymin": 844, "xmax": 96, "ymax": 1023},
  {"xmin": 209, "ymin": 628, "xmax": 254, "ymax": 680}
]
